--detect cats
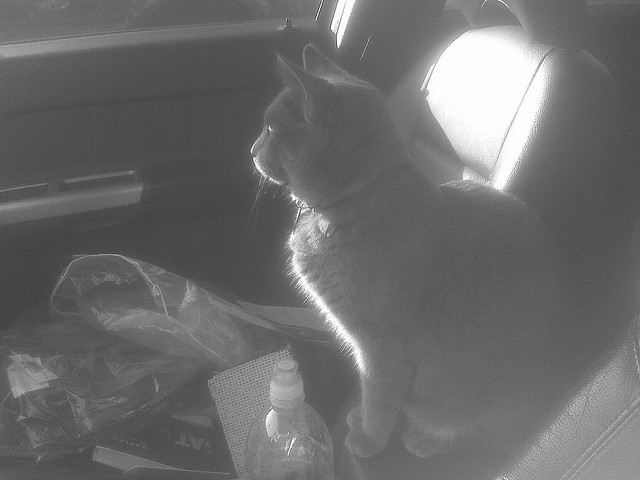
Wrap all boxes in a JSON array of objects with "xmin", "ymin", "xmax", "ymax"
[{"xmin": 249, "ymin": 44, "xmax": 570, "ymax": 461}]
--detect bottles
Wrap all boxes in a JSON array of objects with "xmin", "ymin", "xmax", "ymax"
[{"xmin": 244, "ymin": 360, "xmax": 335, "ymax": 480}]
